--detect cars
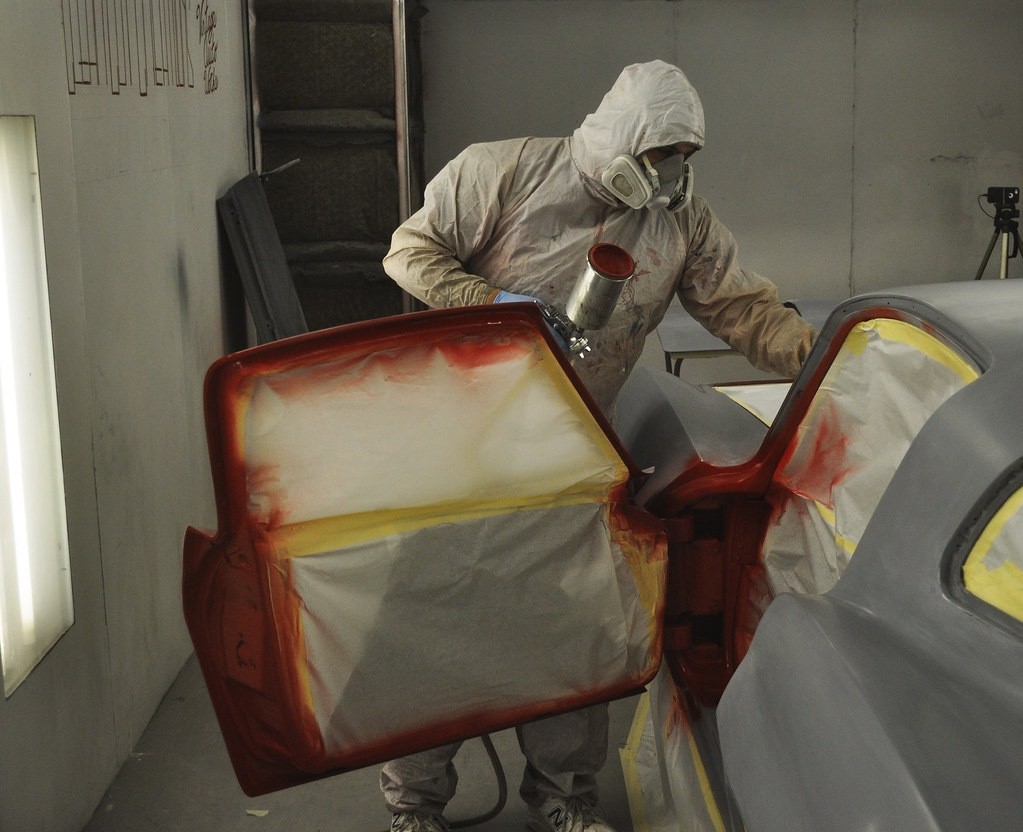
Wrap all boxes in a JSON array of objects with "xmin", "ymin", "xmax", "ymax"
[{"xmin": 183, "ymin": 278, "xmax": 1023, "ymax": 832}]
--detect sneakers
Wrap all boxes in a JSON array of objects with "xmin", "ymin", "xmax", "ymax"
[
  {"xmin": 524, "ymin": 792, "xmax": 616, "ymax": 832},
  {"xmin": 390, "ymin": 811, "xmax": 451, "ymax": 832}
]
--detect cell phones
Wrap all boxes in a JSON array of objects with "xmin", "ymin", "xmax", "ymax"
[{"xmin": 987, "ymin": 186, "xmax": 1019, "ymax": 204}]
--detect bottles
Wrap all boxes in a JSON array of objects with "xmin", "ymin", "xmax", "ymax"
[{"xmin": 565, "ymin": 242, "xmax": 636, "ymax": 334}]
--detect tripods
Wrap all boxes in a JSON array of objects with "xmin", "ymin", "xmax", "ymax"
[{"xmin": 975, "ymin": 203, "xmax": 1023, "ymax": 281}]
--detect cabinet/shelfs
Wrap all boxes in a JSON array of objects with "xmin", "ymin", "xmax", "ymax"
[{"xmin": 241, "ymin": 0, "xmax": 415, "ymax": 316}]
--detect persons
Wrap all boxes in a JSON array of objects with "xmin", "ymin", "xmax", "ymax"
[{"xmin": 379, "ymin": 58, "xmax": 819, "ymax": 832}]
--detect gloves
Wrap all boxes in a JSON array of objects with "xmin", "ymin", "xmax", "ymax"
[{"xmin": 492, "ymin": 290, "xmax": 567, "ymax": 354}]
